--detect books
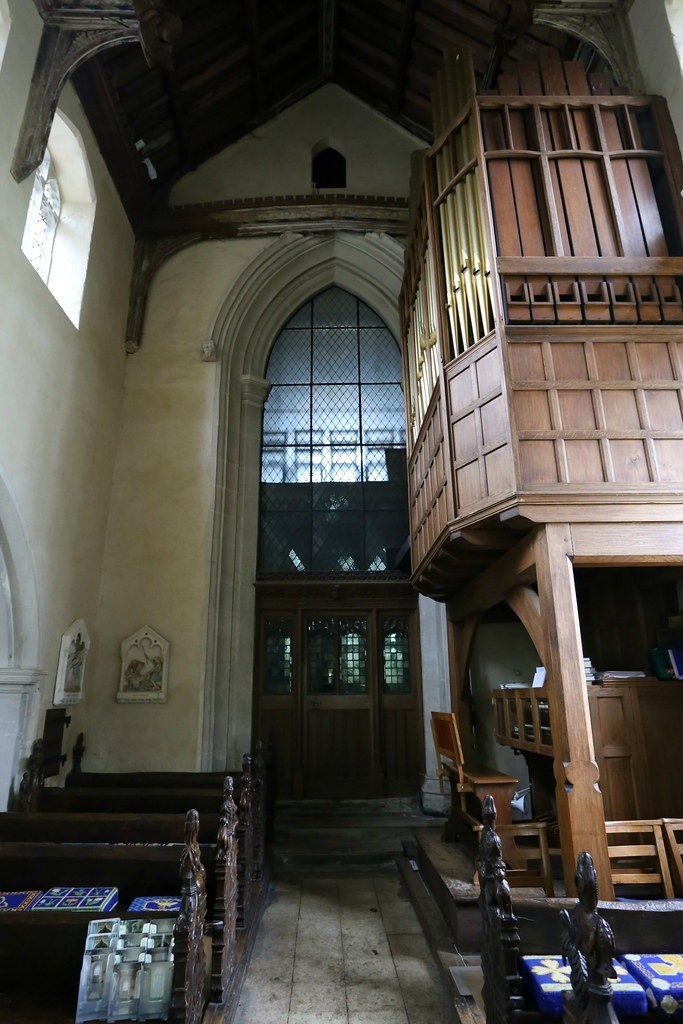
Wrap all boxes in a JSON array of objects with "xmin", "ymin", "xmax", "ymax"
[{"xmin": 584, "ymin": 658, "xmax": 595, "ymax": 685}]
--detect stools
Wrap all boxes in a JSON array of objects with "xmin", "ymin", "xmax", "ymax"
[{"xmin": 473, "ymin": 817, "xmax": 683, "ymax": 899}]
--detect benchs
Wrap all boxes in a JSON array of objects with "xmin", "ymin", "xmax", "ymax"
[{"xmin": 430, "ymin": 711, "xmax": 527, "ymax": 869}]
